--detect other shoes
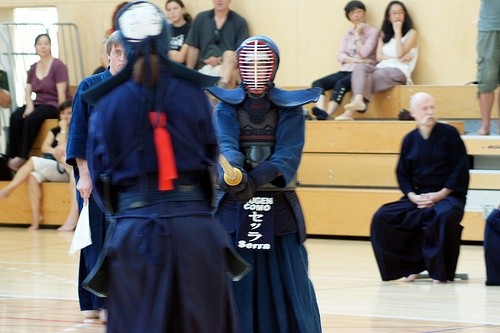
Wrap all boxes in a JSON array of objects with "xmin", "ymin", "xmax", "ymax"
[
  {"xmin": 8, "ymin": 157, "xmax": 27, "ymax": 171},
  {"xmin": 312, "ymin": 106, "xmax": 328, "ymax": 119},
  {"xmin": 344, "ymin": 102, "xmax": 366, "ymax": 110},
  {"xmin": 335, "ymin": 114, "xmax": 353, "ymax": 121}
]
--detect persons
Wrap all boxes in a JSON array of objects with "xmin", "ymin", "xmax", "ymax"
[
  {"xmin": 184, "ymin": 0, "xmax": 249, "ymax": 90},
  {"xmin": 311, "ymin": 0, "xmax": 378, "ymax": 121},
  {"xmin": 164, "ymin": 0, "xmax": 193, "ymax": 65},
  {"xmin": 81, "ymin": 1, "xmax": 251, "ymax": 333},
  {"xmin": 334, "ymin": 0, "xmax": 418, "ymax": 121},
  {"xmin": 56, "ymin": 1, "xmax": 135, "ymax": 324},
  {"xmin": 6, "ymin": 34, "xmax": 72, "ymax": 170},
  {"xmin": 0, "ymin": 70, "xmax": 12, "ymax": 180},
  {"xmin": 0, "ymin": 99, "xmax": 71, "ymax": 232},
  {"xmin": 204, "ymin": 36, "xmax": 322, "ymax": 333},
  {"xmin": 476, "ymin": 0, "xmax": 500, "ymax": 136},
  {"xmin": 371, "ymin": 92, "xmax": 471, "ymax": 284}
]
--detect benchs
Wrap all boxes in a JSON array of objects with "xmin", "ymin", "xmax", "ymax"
[{"xmin": 1, "ymin": 85, "xmax": 500, "ymax": 241}]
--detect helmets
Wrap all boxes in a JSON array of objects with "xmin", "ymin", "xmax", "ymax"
[
  {"xmin": 236, "ymin": 36, "xmax": 280, "ymax": 94},
  {"xmin": 115, "ymin": 0, "xmax": 170, "ymax": 64}
]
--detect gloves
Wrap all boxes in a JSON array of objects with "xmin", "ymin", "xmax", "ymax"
[
  {"xmin": 233, "ymin": 178, "xmax": 257, "ymax": 202},
  {"xmin": 224, "ymin": 166, "xmax": 248, "ymax": 194}
]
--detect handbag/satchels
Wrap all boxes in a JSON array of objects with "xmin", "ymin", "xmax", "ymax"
[
  {"xmin": 0, "ymin": 153, "xmax": 13, "ymax": 182},
  {"xmin": 44, "ymin": 153, "xmax": 66, "ymax": 173}
]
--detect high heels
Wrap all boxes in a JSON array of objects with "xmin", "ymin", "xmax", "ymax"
[{"xmin": 57, "ymin": 222, "xmax": 77, "ymax": 231}]
